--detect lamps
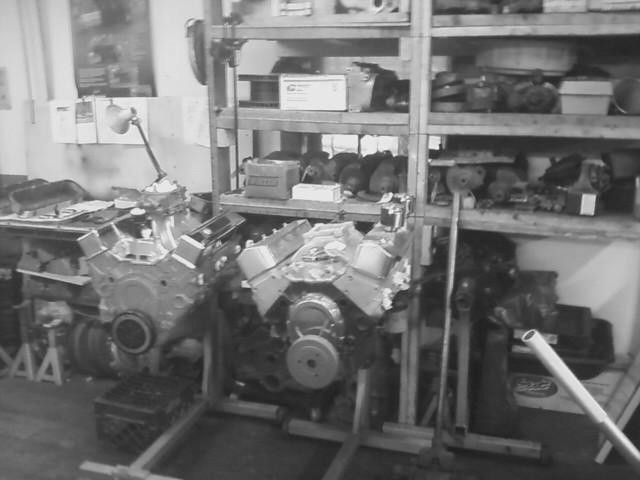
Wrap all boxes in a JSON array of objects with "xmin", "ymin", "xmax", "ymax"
[{"xmin": 103, "ymin": 104, "xmax": 178, "ymax": 193}]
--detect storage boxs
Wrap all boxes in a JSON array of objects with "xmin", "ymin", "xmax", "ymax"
[{"xmin": 9, "ymin": 180, "xmax": 85, "ymax": 218}]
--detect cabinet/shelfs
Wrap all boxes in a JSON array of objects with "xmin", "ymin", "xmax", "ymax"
[
  {"xmin": 206, "ymin": 2, "xmax": 417, "ymax": 30},
  {"xmin": 208, "ymin": 210, "xmax": 405, "ymax": 424},
  {"xmin": 208, "ymin": 35, "xmax": 412, "ymax": 119},
  {"xmin": 419, "ymin": 132, "xmax": 639, "ymax": 228},
  {"xmin": 211, "ymin": 123, "xmax": 417, "ymax": 215},
  {"xmin": 429, "ymin": 2, "xmax": 638, "ymax": 31},
  {"xmin": 418, "ymin": 35, "xmax": 637, "ymax": 127},
  {"xmin": 408, "ymin": 218, "xmax": 638, "ymax": 425}
]
